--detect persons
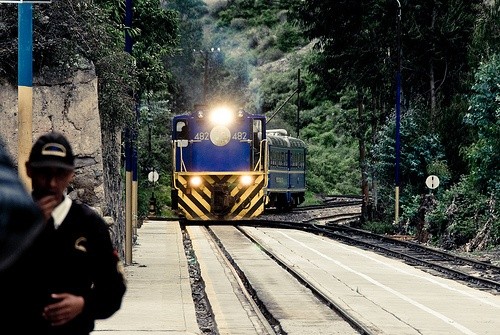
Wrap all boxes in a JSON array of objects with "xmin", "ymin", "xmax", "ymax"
[{"xmin": 0, "ymin": 131, "xmax": 127, "ymax": 335}]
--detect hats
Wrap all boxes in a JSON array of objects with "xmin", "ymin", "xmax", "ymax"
[{"xmin": 27, "ymin": 132, "xmax": 77, "ymax": 172}]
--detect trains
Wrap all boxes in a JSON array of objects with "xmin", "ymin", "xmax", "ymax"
[{"xmin": 171, "ymin": 107, "xmax": 308, "ymax": 221}]
